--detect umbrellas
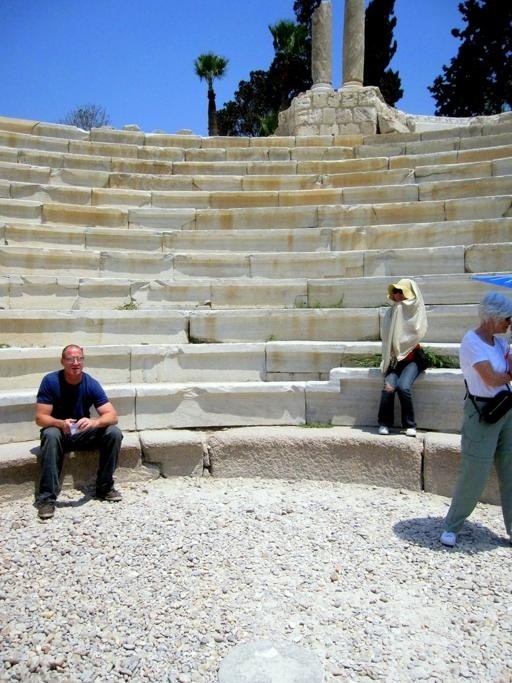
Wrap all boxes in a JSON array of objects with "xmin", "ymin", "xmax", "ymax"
[{"xmin": 467, "ymin": 274, "xmax": 511, "ymax": 290}]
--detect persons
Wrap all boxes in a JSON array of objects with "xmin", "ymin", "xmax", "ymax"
[
  {"xmin": 35, "ymin": 343, "xmax": 124, "ymax": 519},
  {"xmin": 377, "ymin": 278, "xmax": 429, "ymax": 436},
  {"xmin": 439, "ymin": 290, "xmax": 511, "ymax": 546}
]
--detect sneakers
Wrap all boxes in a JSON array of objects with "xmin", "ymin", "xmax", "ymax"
[
  {"xmin": 440, "ymin": 530, "xmax": 457, "ymax": 548},
  {"xmin": 95, "ymin": 488, "xmax": 122, "ymax": 502},
  {"xmin": 37, "ymin": 502, "xmax": 55, "ymax": 518},
  {"xmin": 406, "ymin": 428, "xmax": 417, "ymax": 438},
  {"xmin": 378, "ymin": 425, "xmax": 389, "ymax": 435}
]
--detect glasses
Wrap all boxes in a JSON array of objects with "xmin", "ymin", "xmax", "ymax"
[
  {"xmin": 391, "ymin": 288, "xmax": 403, "ymax": 295},
  {"xmin": 505, "ymin": 316, "xmax": 511, "ymax": 321}
]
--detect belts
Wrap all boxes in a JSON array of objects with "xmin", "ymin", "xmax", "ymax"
[{"xmin": 469, "ymin": 394, "xmax": 490, "ymax": 403}]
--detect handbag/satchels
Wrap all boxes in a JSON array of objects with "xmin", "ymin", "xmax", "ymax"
[
  {"xmin": 479, "ymin": 389, "xmax": 512, "ymax": 424},
  {"xmin": 411, "ymin": 347, "xmax": 432, "ymax": 371}
]
